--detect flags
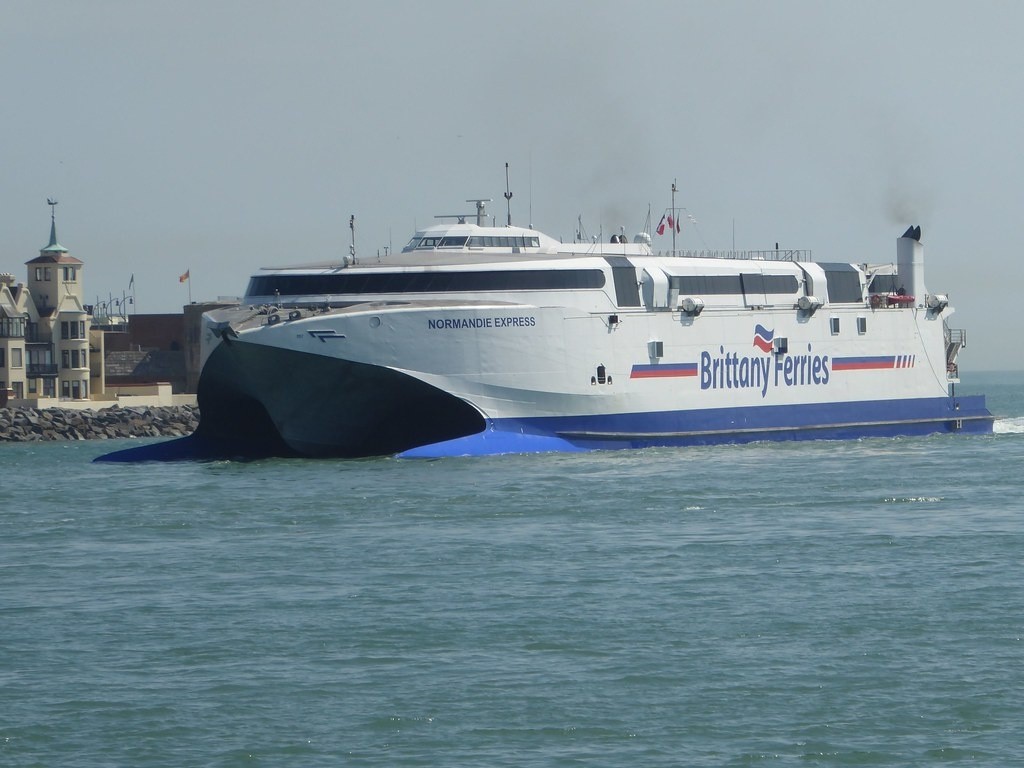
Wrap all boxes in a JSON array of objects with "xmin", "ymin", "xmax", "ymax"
[
  {"xmin": 179, "ymin": 271, "xmax": 189, "ymax": 282},
  {"xmin": 128, "ymin": 275, "xmax": 134, "ymax": 290},
  {"xmin": 657, "ymin": 215, "xmax": 680, "ymax": 235}
]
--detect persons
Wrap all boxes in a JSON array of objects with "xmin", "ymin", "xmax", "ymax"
[{"xmin": 897, "ymin": 284, "xmax": 906, "ymax": 308}]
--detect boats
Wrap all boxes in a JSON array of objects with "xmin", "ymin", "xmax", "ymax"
[{"xmin": 198, "ymin": 160, "xmax": 997, "ymax": 465}]
